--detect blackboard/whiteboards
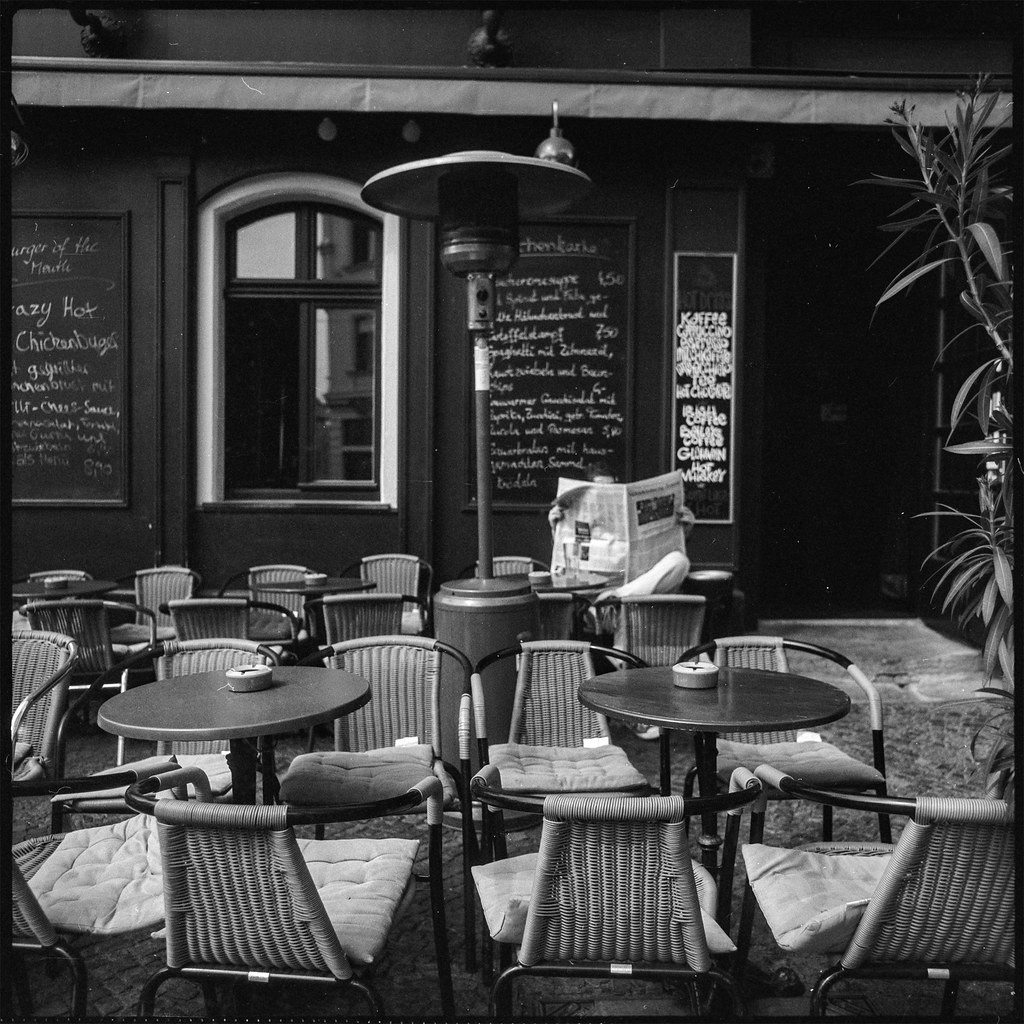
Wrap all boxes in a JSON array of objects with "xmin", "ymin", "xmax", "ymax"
[
  {"xmin": 460, "ymin": 213, "xmax": 640, "ymax": 515},
  {"xmin": 665, "ymin": 251, "xmax": 740, "ymax": 525},
  {"xmin": 11, "ymin": 208, "xmax": 134, "ymax": 509}
]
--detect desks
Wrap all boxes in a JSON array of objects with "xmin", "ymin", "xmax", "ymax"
[
  {"xmin": 524, "ymin": 571, "xmax": 609, "ymax": 593},
  {"xmin": 253, "ymin": 579, "xmax": 377, "ymax": 638},
  {"xmin": 12, "ymin": 578, "xmax": 118, "ymax": 616},
  {"xmin": 574, "ymin": 661, "xmax": 851, "ymax": 1012},
  {"xmin": 95, "ymin": 666, "xmax": 373, "ymax": 811}
]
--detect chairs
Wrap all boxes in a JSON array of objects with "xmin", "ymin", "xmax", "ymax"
[{"xmin": 7, "ymin": 550, "xmax": 1020, "ymax": 1020}]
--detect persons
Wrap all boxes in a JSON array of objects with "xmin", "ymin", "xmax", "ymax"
[{"xmin": 548, "ymin": 462, "xmax": 695, "ymax": 631}]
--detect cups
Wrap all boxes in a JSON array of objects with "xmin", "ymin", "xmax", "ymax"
[{"xmin": 563, "ymin": 542, "xmax": 580, "ymax": 581}]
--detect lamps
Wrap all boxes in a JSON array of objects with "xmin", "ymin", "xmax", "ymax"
[{"xmin": 528, "ymin": 99, "xmax": 584, "ymax": 168}]
